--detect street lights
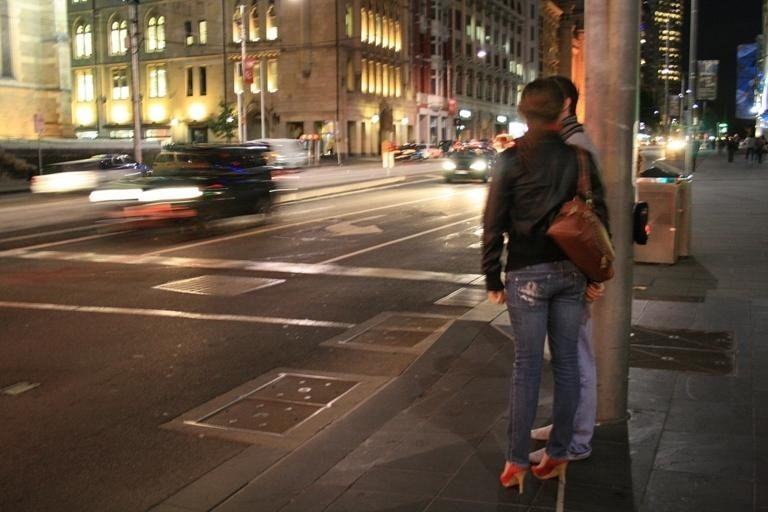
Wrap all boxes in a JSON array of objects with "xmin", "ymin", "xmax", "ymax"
[{"xmin": 447, "ymin": 49, "xmax": 487, "ymax": 140}]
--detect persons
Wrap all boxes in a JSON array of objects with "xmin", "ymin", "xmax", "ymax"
[
  {"xmin": 526, "ymin": 77, "xmax": 615, "ymax": 467},
  {"xmin": 691, "ymin": 132, "xmax": 768, "ymax": 172},
  {"xmin": 481, "ymin": 76, "xmax": 616, "ymax": 488}
]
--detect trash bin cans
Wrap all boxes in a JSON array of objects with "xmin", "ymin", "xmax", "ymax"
[
  {"xmin": 382, "ymin": 151, "xmax": 395, "ymax": 169},
  {"xmin": 633, "ymin": 160, "xmax": 693, "ymax": 265}
]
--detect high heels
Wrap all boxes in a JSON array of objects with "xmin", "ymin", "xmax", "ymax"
[
  {"xmin": 500, "ymin": 464, "xmax": 529, "ymax": 495},
  {"xmin": 531, "ymin": 458, "xmax": 569, "ymax": 485}
]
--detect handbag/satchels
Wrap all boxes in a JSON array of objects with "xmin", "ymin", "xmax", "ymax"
[{"xmin": 549, "ymin": 197, "xmax": 616, "ymax": 283}]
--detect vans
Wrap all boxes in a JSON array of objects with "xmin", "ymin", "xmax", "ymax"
[{"xmin": 240, "ymin": 138, "xmax": 308, "ymax": 177}]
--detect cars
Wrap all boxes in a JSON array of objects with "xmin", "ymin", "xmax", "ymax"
[
  {"xmin": 89, "ymin": 142, "xmax": 275, "ymax": 243},
  {"xmin": 393, "ymin": 133, "xmax": 516, "ymax": 163},
  {"xmin": 443, "ymin": 145, "xmax": 497, "ymax": 183},
  {"xmin": 27, "ymin": 154, "xmax": 152, "ymax": 199}
]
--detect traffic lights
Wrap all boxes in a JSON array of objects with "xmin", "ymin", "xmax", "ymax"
[
  {"xmin": 300, "ymin": 134, "xmax": 319, "ymax": 141},
  {"xmin": 449, "ymin": 98, "xmax": 456, "ymax": 116}
]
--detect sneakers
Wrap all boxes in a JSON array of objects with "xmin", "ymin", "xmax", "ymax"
[
  {"xmin": 530, "ymin": 424, "xmax": 553, "ymax": 440},
  {"xmin": 529, "ymin": 448, "xmax": 592, "ymax": 462}
]
